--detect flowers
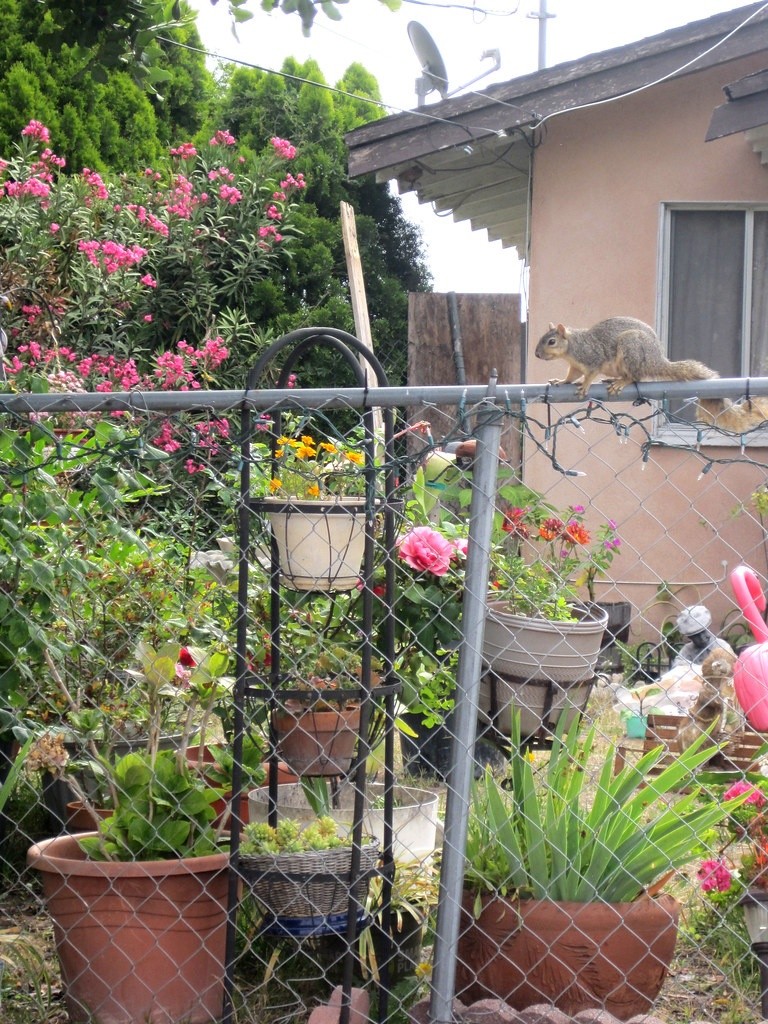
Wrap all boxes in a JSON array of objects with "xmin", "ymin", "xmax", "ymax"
[
  {"xmin": 273, "ymin": 437, "xmax": 364, "ymax": 498},
  {"xmin": 467, "ymin": 487, "xmax": 622, "ymax": 622}
]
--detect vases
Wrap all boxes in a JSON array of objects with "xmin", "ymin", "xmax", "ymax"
[
  {"xmin": 478, "ymin": 601, "xmax": 607, "ymax": 744},
  {"xmin": 258, "ymin": 495, "xmax": 368, "ymax": 593}
]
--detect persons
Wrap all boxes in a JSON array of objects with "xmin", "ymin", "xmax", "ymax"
[{"xmin": 672, "ymin": 605, "xmax": 737, "ymax": 669}]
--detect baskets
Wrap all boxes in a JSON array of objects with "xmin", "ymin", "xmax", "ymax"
[{"xmin": 238, "ymin": 834, "xmax": 379, "ymax": 916}]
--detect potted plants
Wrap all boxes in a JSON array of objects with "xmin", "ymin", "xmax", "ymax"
[
  {"xmin": 64, "ymin": 538, "xmax": 200, "ymax": 783},
  {"xmin": 249, "ymin": 818, "xmax": 378, "ymax": 938},
  {"xmin": 54, "ymin": 709, "xmax": 122, "ymax": 829},
  {"xmin": 446, "ymin": 716, "xmax": 754, "ymax": 1015},
  {"xmin": 247, "ymin": 708, "xmax": 439, "ymax": 935},
  {"xmin": 261, "ymin": 606, "xmax": 363, "ymax": 775},
  {"xmin": 30, "ymin": 752, "xmax": 230, "ymax": 1024},
  {"xmin": 178, "ymin": 685, "xmax": 311, "ymax": 829}
]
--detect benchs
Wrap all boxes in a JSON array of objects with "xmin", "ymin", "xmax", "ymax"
[{"xmin": 608, "ymin": 714, "xmax": 767, "ymax": 807}]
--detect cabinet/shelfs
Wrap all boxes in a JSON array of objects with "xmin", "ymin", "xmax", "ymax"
[{"xmin": 224, "ymin": 326, "xmax": 399, "ymax": 1024}]
